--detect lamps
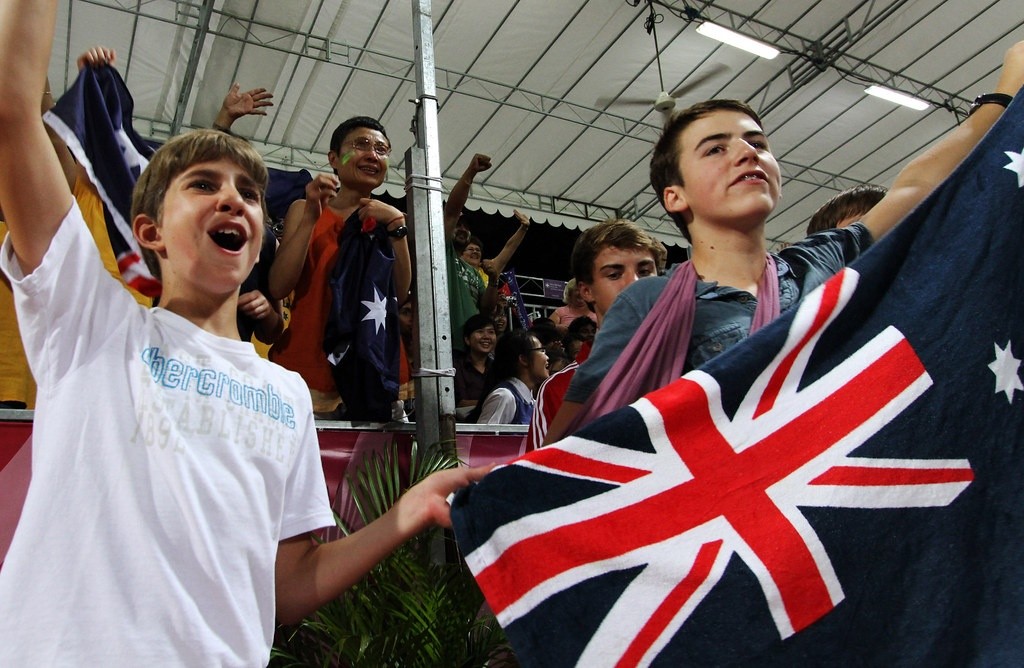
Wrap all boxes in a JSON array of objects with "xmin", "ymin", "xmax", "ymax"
[
  {"xmin": 695, "ymin": 19, "xmax": 781, "ymax": 60},
  {"xmin": 863, "ymin": 84, "xmax": 932, "ymax": 111}
]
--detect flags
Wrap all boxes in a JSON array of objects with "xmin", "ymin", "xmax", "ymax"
[
  {"xmin": 449, "ymin": 86, "xmax": 1024, "ymax": 668},
  {"xmin": 44, "ymin": 63, "xmax": 162, "ymax": 298}
]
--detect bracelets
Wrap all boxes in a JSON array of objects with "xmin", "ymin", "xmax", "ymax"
[
  {"xmin": 43, "ymin": 92, "xmax": 51, "ymax": 94},
  {"xmin": 389, "ymin": 215, "xmax": 404, "ymax": 224},
  {"xmin": 212, "ymin": 122, "xmax": 220, "ymax": 129},
  {"xmin": 969, "ymin": 93, "xmax": 1013, "ymax": 117},
  {"xmin": 388, "ymin": 226, "xmax": 407, "ymax": 239}
]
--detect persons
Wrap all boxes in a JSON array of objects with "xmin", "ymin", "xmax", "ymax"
[
  {"xmin": 1, "ymin": 48, "xmax": 290, "ymax": 410},
  {"xmin": 0, "ymin": 0, "xmax": 496, "ymax": 668},
  {"xmin": 542, "ymin": 40, "xmax": 1024, "ymax": 453},
  {"xmin": 807, "ymin": 185, "xmax": 888, "ymax": 237},
  {"xmin": 525, "ymin": 219, "xmax": 666, "ymax": 452},
  {"xmin": 271, "ymin": 116, "xmax": 411, "ymax": 422},
  {"xmin": 475, "ymin": 332, "xmax": 549, "ymax": 425},
  {"xmin": 398, "ymin": 153, "xmax": 599, "ymax": 423}
]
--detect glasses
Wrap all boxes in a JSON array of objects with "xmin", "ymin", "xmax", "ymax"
[
  {"xmin": 531, "ymin": 346, "xmax": 547, "ymax": 353},
  {"xmin": 342, "ymin": 136, "xmax": 392, "ymax": 157}
]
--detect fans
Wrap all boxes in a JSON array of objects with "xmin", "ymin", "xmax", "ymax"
[{"xmin": 593, "ymin": 0, "xmax": 732, "ymax": 124}]
